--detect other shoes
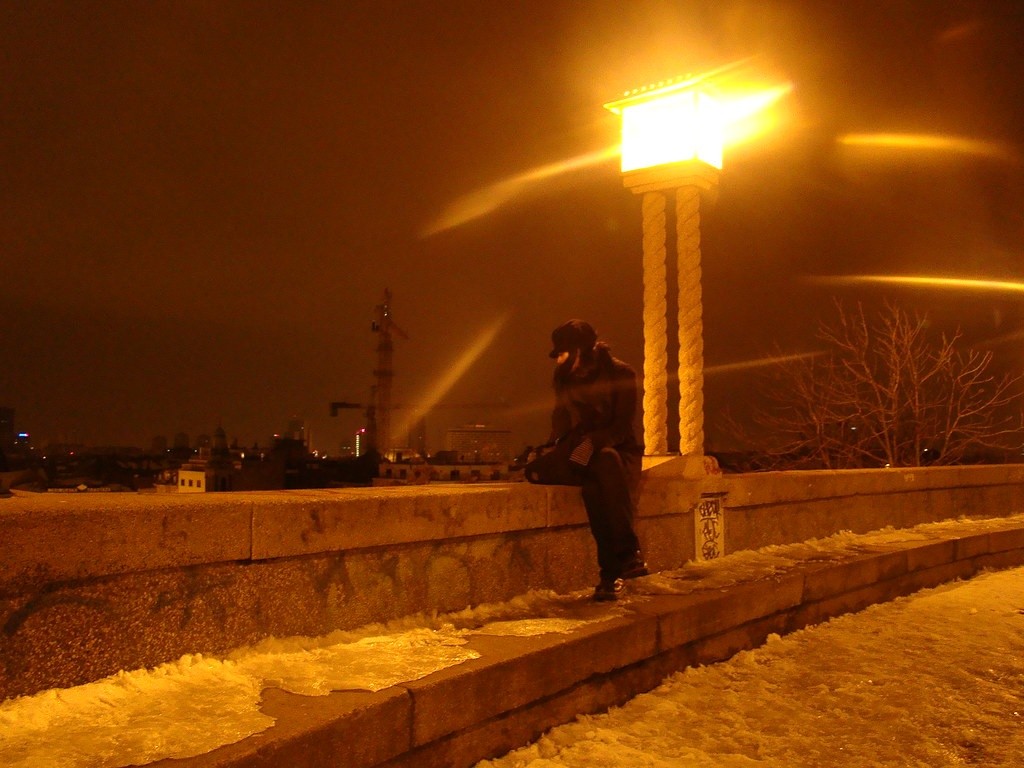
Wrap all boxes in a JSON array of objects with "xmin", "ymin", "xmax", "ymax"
[
  {"xmin": 622, "ymin": 549, "xmax": 648, "ymax": 579},
  {"xmin": 594, "ymin": 576, "xmax": 626, "ymax": 600}
]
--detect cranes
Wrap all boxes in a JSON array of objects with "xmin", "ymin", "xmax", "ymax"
[{"xmin": 330, "ymin": 288, "xmax": 509, "ymax": 457}]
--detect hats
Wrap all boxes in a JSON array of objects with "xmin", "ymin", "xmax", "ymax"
[{"xmin": 549, "ymin": 319, "xmax": 596, "ymax": 357}]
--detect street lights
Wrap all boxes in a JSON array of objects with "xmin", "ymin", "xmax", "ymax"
[{"xmin": 602, "ymin": 62, "xmax": 781, "ymax": 456}]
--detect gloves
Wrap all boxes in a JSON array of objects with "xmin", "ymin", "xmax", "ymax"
[{"xmin": 569, "ymin": 438, "xmax": 595, "ymax": 472}]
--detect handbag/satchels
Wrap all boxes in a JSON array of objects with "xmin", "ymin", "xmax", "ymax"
[{"xmin": 524, "ymin": 437, "xmax": 579, "ymax": 485}]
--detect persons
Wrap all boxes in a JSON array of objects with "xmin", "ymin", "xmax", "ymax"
[{"xmin": 549, "ymin": 319, "xmax": 649, "ymax": 600}]
